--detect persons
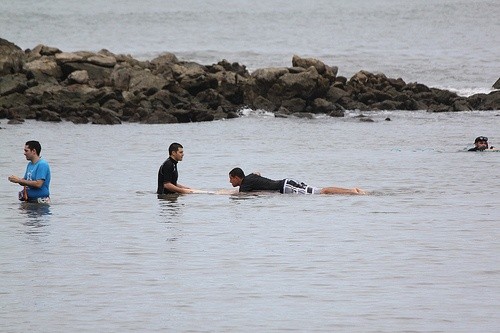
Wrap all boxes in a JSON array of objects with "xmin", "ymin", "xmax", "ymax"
[
  {"xmin": 8, "ymin": 140, "xmax": 51, "ymax": 203},
  {"xmin": 157, "ymin": 143, "xmax": 193, "ymax": 194},
  {"xmin": 229, "ymin": 168, "xmax": 368, "ymax": 195},
  {"xmin": 468, "ymin": 136, "xmax": 500, "ymax": 152}
]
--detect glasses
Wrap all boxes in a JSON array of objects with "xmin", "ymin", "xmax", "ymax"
[{"xmin": 479, "ymin": 137, "xmax": 487, "ymax": 140}]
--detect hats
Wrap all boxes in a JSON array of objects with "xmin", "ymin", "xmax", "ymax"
[{"xmin": 475, "ymin": 136, "xmax": 487, "ymax": 143}]
